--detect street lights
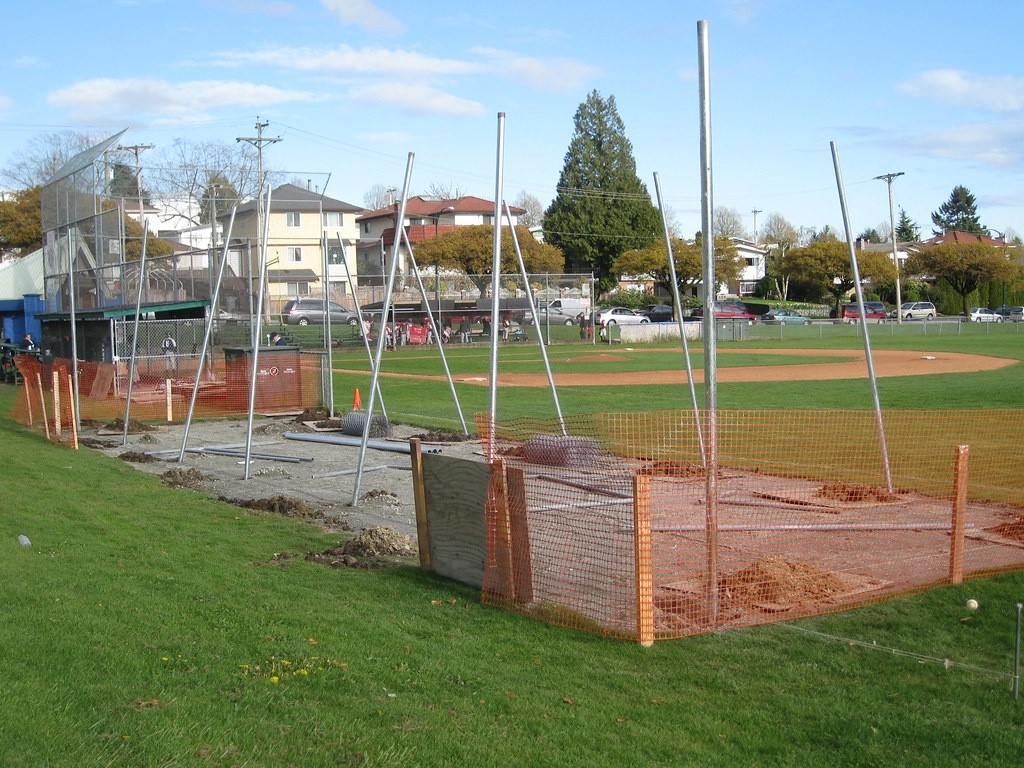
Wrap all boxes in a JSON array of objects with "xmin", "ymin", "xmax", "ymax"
[
  {"xmin": 982, "ymin": 229, "xmax": 1006, "ymax": 306},
  {"xmin": 435, "ymin": 207, "xmax": 454, "ymax": 300}
]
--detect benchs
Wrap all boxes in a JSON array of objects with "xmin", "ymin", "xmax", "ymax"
[
  {"xmin": 216, "ymin": 322, "xmax": 527, "ymax": 347},
  {"xmin": 0, "ymin": 343, "xmax": 43, "ymax": 386}
]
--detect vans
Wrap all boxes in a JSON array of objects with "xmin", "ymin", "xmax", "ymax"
[
  {"xmin": 542, "ymin": 298, "xmax": 590, "ymax": 323},
  {"xmin": 280, "ymin": 298, "xmax": 359, "ymax": 326},
  {"xmin": 890, "ymin": 302, "xmax": 936, "ymax": 321}
]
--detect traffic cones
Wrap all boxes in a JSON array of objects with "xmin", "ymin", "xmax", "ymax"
[{"xmin": 351, "ymin": 389, "xmax": 364, "ymax": 411}]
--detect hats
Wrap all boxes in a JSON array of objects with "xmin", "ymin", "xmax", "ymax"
[{"xmin": 270, "ymin": 332, "xmax": 277, "ymax": 340}]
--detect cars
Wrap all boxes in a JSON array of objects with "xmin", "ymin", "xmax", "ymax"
[
  {"xmin": 1009, "ymin": 307, "xmax": 1024, "ymax": 323},
  {"xmin": 634, "ymin": 304, "xmax": 674, "ymax": 323},
  {"xmin": 691, "ymin": 303, "xmax": 757, "ymax": 325},
  {"xmin": 992, "ymin": 306, "xmax": 1011, "ymax": 320},
  {"xmin": 863, "ymin": 301, "xmax": 886, "ymax": 314},
  {"xmin": 761, "ymin": 309, "xmax": 812, "ymax": 326},
  {"xmin": 959, "ymin": 307, "xmax": 1003, "ymax": 323},
  {"xmin": 214, "ymin": 309, "xmax": 242, "ymax": 325},
  {"xmin": 589, "ymin": 307, "xmax": 651, "ymax": 326},
  {"xmin": 523, "ymin": 308, "xmax": 577, "ymax": 326}
]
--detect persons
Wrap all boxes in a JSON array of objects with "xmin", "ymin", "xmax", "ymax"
[
  {"xmin": 360, "ymin": 319, "xmax": 374, "ymax": 339},
  {"xmin": 580, "ymin": 313, "xmax": 594, "ymax": 338},
  {"xmin": 161, "ymin": 332, "xmax": 176, "ymax": 373},
  {"xmin": 436, "ymin": 317, "xmax": 439, "ymax": 330},
  {"xmin": 600, "ymin": 320, "xmax": 606, "ymax": 341},
  {"xmin": 270, "ymin": 332, "xmax": 287, "ymax": 346},
  {"xmin": 460, "ymin": 316, "xmax": 470, "ymax": 343},
  {"xmin": 127, "ymin": 335, "xmax": 140, "ymax": 384},
  {"xmin": 384, "ymin": 318, "xmax": 432, "ymax": 352},
  {"xmin": 483, "ymin": 316, "xmax": 490, "ymax": 333},
  {"xmin": 24, "ymin": 334, "xmax": 33, "ymax": 350},
  {"xmin": 502, "ymin": 316, "xmax": 509, "ymax": 342}
]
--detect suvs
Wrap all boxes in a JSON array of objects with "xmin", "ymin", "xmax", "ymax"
[{"xmin": 830, "ymin": 303, "xmax": 887, "ymax": 325}]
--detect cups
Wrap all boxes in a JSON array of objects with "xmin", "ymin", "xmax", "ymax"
[
  {"xmin": 45, "ymin": 349, "xmax": 50, "ymax": 356},
  {"xmin": 28, "ymin": 345, "xmax": 32, "ymax": 351}
]
